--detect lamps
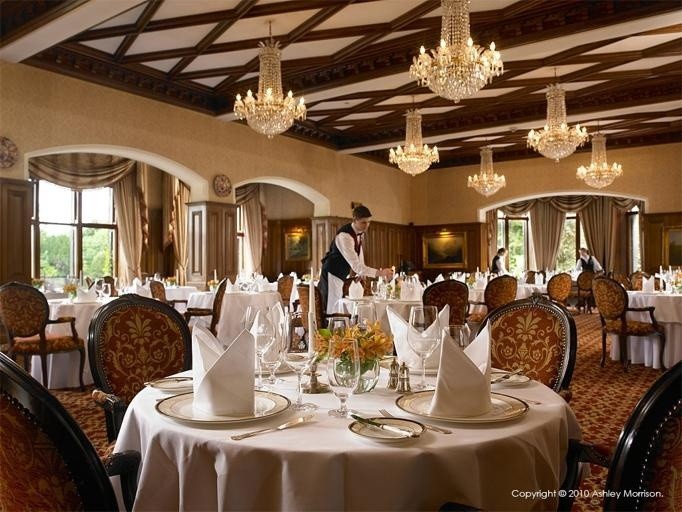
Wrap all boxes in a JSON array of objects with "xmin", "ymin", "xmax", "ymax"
[
  {"xmin": 387, "ymin": 94, "xmax": 440, "ymax": 177},
  {"xmin": 232, "ymin": 17, "xmax": 310, "ymax": 139},
  {"xmin": 524, "ymin": 65, "xmax": 593, "ymax": 163},
  {"xmin": 467, "ymin": 134, "xmax": 508, "ymax": 197},
  {"xmin": 574, "ymin": 113, "xmax": 624, "ymax": 188},
  {"xmin": 406, "ymin": 0, "xmax": 505, "ymax": 107}
]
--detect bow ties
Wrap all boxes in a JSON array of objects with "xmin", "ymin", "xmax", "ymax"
[{"xmin": 357, "ymin": 233, "xmax": 363, "ymax": 235}]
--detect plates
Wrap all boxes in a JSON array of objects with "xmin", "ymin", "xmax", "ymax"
[
  {"xmin": 396, "ymin": 389, "xmax": 528, "ymax": 422},
  {"xmin": 146, "ymin": 376, "xmax": 194, "ymax": 391},
  {"xmin": 490, "ymin": 371, "xmax": 530, "ymax": 387},
  {"xmin": 155, "ymin": 389, "xmax": 292, "ymax": 423},
  {"xmin": 254, "ymin": 353, "xmax": 305, "ymax": 374},
  {"xmin": 348, "ymin": 417, "xmax": 426, "ymax": 443}
]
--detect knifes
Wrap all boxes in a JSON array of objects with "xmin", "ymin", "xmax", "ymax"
[
  {"xmin": 491, "ymin": 368, "xmax": 523, "ymax": 384},
  {"xmin": 231, "ymin": 415, "xmax": 315, "ymax": 440},
  {"xmin": 350, "ymin": 414, "xmax": 419, "ymax": 438}
]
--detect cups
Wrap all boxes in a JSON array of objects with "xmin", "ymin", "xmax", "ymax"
[{"xmin": 446, "ymin": 324, "xmax": 464, "ymax": 349}]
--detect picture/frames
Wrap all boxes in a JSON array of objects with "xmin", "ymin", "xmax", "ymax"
[
  {"xmin": 422, "ymin": 231, "xmax": 468, "ymax": 271},
  {"xmin": 283, "ymin": 232, "xmax": 311, "ymax": 263},
  {"xmin": 660, "ymin": 225, "xmax": 681, "ymax": 267}
]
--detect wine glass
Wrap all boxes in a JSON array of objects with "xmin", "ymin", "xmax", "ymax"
[
  {"xmin": 326, "ymin": 338, "xmax": 361, "ymax": 417},
  {"xmin": 407, "ymin": 306, "xmax": 440, "ymax": 391},
  {"xmin": 245, "ymin": 305, "xmax": 275, "ymax": 388},
  {"xmin": 95, "ymin": 278, "xmax": 105, "ymax": 300},
  {"xmin": 102, "ymin": 284, "xmax": 111, "ymax": 300},
  {"xmin": 262, "ymin": 339, "xmax": 283, "ymax": 384},
  {"xmin": 281, "ymin": 311, "xmax": 317, "ymax": 412},
  {"xmin": 405, "ymin": 276, "xmax": 416, "ymax": 298},
  {"xmin": 370, "ymin": 280, "xmax": 380, "ymax": 299}
]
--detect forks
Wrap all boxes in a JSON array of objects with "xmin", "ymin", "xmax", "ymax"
[{"xmin": 378, "ymin": 408, "xmax": 452, "ymax": 434}]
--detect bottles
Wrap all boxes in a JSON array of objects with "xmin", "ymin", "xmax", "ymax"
[
  {"xmin": 395, "ymin": 362, "xmax": 412, "ymax": 394},
  {"xmin": 386, "ymin": 357, "xmax": 399, "ymax": 390}
]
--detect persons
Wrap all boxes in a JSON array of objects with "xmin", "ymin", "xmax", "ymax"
[
  {"xmin": 575, "ymin": 248, "xmax": 607, "ymax": 309},
  {"xmin": 317, "ymin": 206, "xmax": 393, "ymax": 329},
  {"xmin": 492, "ymin": 248, "xmax": 509, "ymax": 274}
]
